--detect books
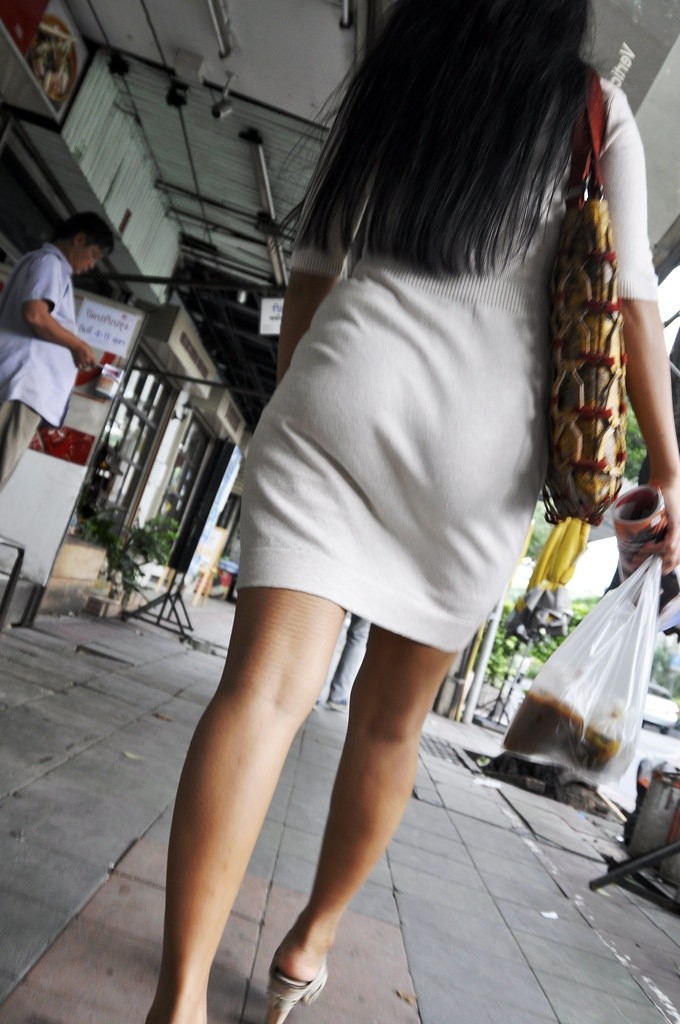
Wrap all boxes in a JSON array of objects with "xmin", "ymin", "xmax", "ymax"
[{"xmin": 612, "ymin": 486, "xmax": 669, "ymax": 606}]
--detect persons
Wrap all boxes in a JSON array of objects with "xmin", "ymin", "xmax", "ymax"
[
  {"xmin": 142, "ymin": 0, "xmax": 677, "ymax": 1024},
  {"xmin": 0, "ymin": 211, "xmax": 113, "ymax": 497}
]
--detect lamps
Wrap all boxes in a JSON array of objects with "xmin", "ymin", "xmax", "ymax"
[
  {"xmin": 211, "ymin": 71, "xmax": 238, "ymax": 119},
  {"xmin": 170, "ymin": 399, "xmax": 193, "ymax": 424}
]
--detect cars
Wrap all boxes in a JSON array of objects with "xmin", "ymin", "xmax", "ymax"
[{"xmin": 639, "ymin": 683, "xmax": 679, "ymax": 736}]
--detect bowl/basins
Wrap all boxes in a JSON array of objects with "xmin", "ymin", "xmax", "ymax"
[{"xmin": 31, "ymin": 14, "xmax": 78, "ymax": 104}]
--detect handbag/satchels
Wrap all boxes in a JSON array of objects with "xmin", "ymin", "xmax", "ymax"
[
  {"xmin": 502, "ymin": 555, "xmax": 660, "ymax": 784},
  {"xmin": 543, "ymin": 69, "xmax": 630, "ymax": 525}
]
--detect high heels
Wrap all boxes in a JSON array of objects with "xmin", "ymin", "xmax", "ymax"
[{"xmin": 264, "ymin": 948, "xmax": 329, "ymax": 1024}]
